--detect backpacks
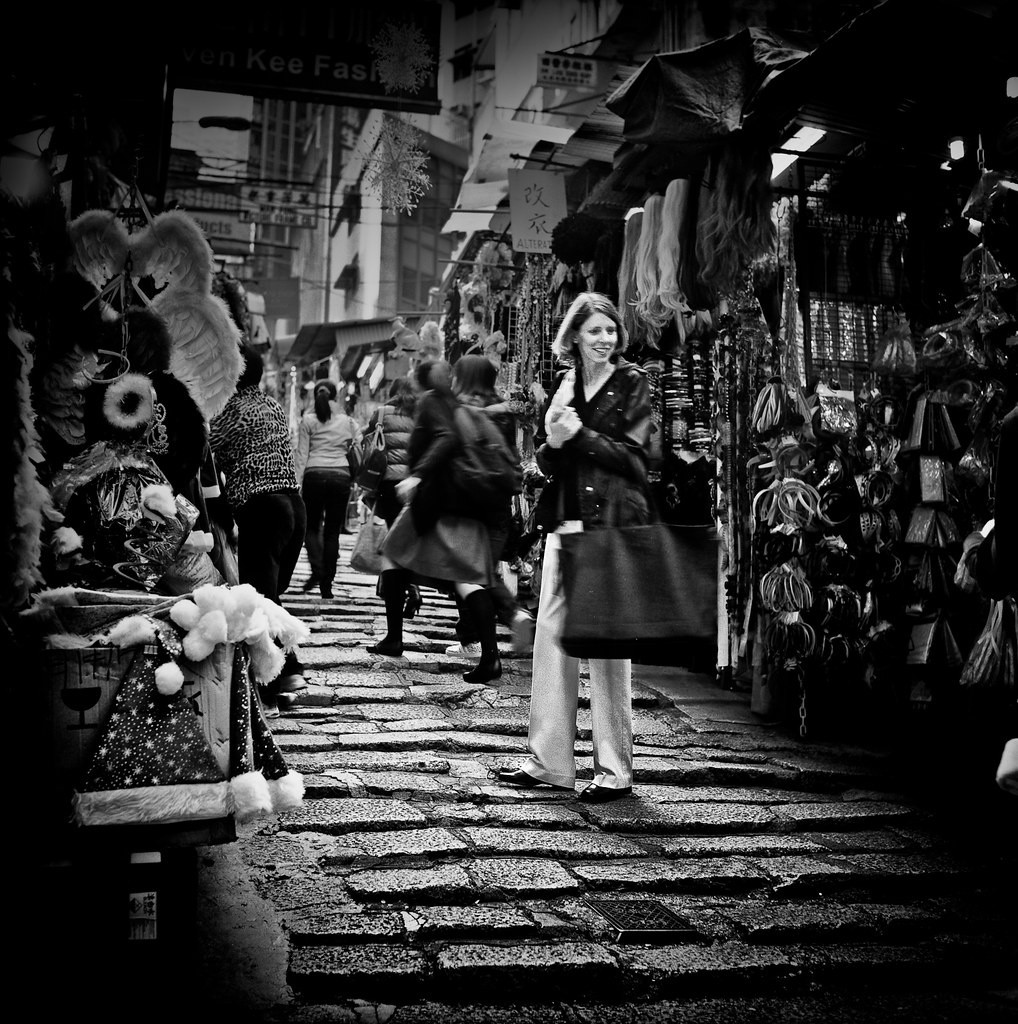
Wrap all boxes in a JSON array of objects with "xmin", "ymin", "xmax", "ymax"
[{"xmin": 417, "ymin": 389, "xmax": 524, "ymax": 495}]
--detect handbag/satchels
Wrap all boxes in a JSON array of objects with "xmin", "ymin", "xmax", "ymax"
[
  {"xmin": 350, "ymin": 502, "xmax": 389, "ymax": 575},
  {"xmin": 559, "ymin": 449, "xmax": 719, "ymax": 660},
  {"xmin": 347, "ymin": 417, "xmax": 364, "ymax": 483},
  {"xmin": 360, "ymin": 406, "xmax": 393, "ymax": 489}
]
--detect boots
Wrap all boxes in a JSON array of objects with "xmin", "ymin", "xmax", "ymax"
[
  {"xmin": 463, "ymin": 589, "xmax": 502, "ymax": 682},
  {"xmin": 366, "ymin": 568, "xmax": 403, "ymax": 656}
]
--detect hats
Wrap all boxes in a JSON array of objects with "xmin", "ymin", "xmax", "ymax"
[{"xmin": 79, "ymin": 584, "xmax": 310, "ymax": 827}]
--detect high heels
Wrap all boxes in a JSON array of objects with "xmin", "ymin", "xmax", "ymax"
[
  {"xmin": 499, "ymin": 769, "xmax": 575, "ymax": 791},
  {"xmin": 579, "ymin": 783, "xmax": 632, "ymax": 803},
  {"xmin": 403, "ymin": 584, "xmax": 421, "ymax": 619}
]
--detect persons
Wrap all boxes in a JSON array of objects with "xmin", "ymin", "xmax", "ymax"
[
  {"xmin": 496, "ymin": 292, "xmax": 658, "ymax": 805},
  {"xmin": 296, "ymin": 378, "xmax": 364, "ymax": 601},
  {"xmin": 203, "ymin": 345, "xmax": 306, "ymax": 717},
  {"xmin": 360, "ymin": 349, "xmax": 536, "ymax": 684}
]
